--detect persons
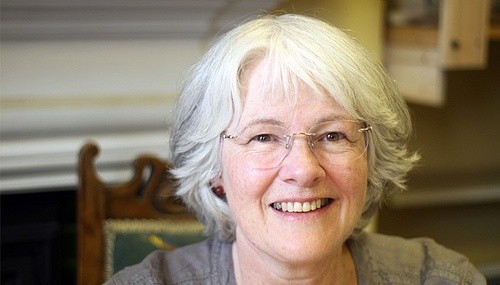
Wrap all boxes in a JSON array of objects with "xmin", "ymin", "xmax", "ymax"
[{"xmin": 100, "ymin": 11, "xmax": 487, "ymax": 285}]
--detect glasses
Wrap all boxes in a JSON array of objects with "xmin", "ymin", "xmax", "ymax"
[{"xmin": 221, "ymin": 119, "xmax": 372, "ymax": 171}]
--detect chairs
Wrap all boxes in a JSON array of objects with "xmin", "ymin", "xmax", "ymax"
[{"xmin": 77, "ymin": 143, "xmax": 208, "ymax": 285}]
